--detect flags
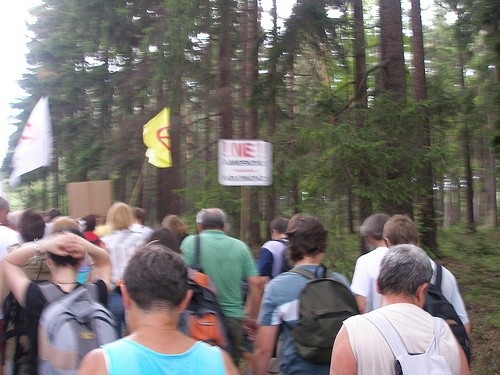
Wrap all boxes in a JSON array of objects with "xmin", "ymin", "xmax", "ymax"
[
  {"xmin": 142, "ymin": 106, "xmax": 174, "ymax": 168},
  {"xmin": 9, "ymin": 94, "xmax": 52, "ymax": 187}
]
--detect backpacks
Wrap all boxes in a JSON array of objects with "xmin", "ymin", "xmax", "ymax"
[
  {"xmin": 283, "ymin": 263, "xmax": 360, "ymax": 366},
  {"xmin": 421, "ymin": 264, "xmax": 472, "ymax": 370},
  {"xmin": 181, "ymin": 234, "xmax": 239, "ymax": 365},
  {"xmin": 34, "ymin": 280, "xmax": 117, "ymax": 375},
  {"xmin": 361, "ymin": 309, "xmax": 452, "ymax": 375},
  {"xmin": 271, "ymin": 236, "xmax": 295, "ymax": 276}
]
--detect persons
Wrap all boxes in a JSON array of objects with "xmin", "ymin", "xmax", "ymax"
[
  {"xmin": 0, "ymin": 198, "xmax": 22, "ymax": 321},
  {"xmin": 328, "ymin": 245, "xmax": 471, "ymax": 375},
  {"xmin": 252, "ymin": 214, "xmax": 359, "ymax": 374},
  {"xmin": 74, "ymin": 243, "xmax": 242, "ymax": 375},
  {"xmin": 40, "ymin": 204, "xmax": 148, "ymax": 248},
  {"xmin": 179, "ymin": 208, "xmax": 260, "ymax": 366},
  {"xmin": 1, "ymin": 227, "xmax": 119, "ymax": 374},
  {"xmin": 101, "ymin": 201, "xmax": 148, "ymax": 341},
  {"xmin": 4, "ymin": 211, "xmax": 54, "ymax": 374},
  {"xmin": 110, "ymin": 229, "xmax": 240, "ymax": 364},
  {"xmin": 351, "ymin": 213, "xmax": 397, "ymax": 315},
  {"xmin": 381, "ymin": 216, "xmax": 471, "ymax": 364},
  {"xmin": 245, "ymin": 218, "xmax": 292, "ymax": 374},
  {"xmin": 160, "ymin": 214, "xmax": 190, "ymax": 243}
]
